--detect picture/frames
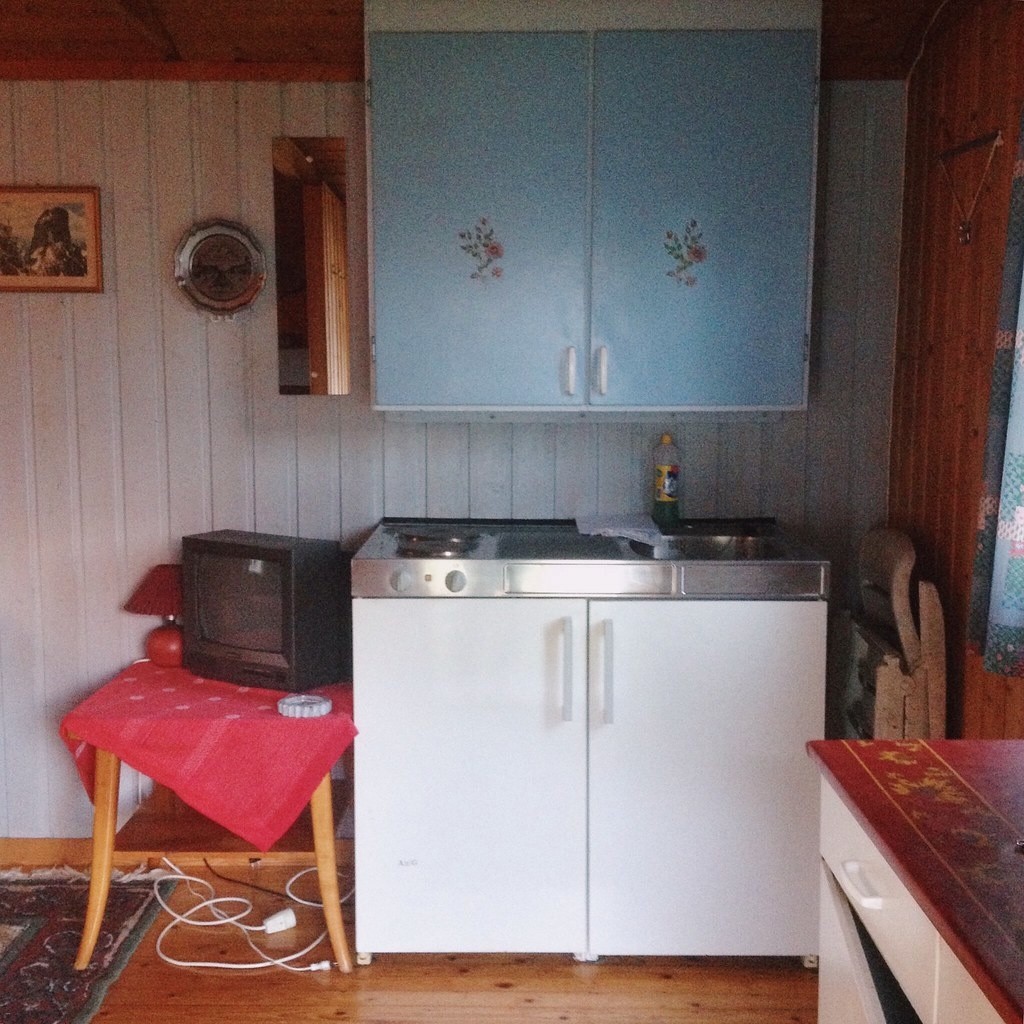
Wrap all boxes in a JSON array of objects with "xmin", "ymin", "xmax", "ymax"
[{"xmin": 0, "ymin": 186, "xmax": 102, "ymax": 294}]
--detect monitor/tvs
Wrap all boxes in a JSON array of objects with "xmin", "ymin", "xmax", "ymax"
[{"xmin": 182, "ymin": 529, "xmax": 353, "ymax": 693}]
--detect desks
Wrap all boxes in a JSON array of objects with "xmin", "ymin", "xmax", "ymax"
[{"xmin": 73, "ymin": 657, "xmax": 353, "ymax": 974}]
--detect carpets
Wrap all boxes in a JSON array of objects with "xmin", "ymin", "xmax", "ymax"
[{"xmin": 0, "ymin": 864, "xmax": 176, "ymax": 1024}]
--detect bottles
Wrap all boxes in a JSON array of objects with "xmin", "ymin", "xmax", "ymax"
[{"xmin": 653, "ymin": 435, "xmax": 682, "ymax": 529}]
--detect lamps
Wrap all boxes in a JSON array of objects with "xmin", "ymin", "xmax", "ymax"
[{"xmin": 123, "ymin": 563, "xmax": 184, "ymax": 668}]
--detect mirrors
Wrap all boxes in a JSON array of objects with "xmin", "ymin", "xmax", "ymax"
[{"xmin": 272, "ymin": 136, "xmax": 350, "ymax": 396}]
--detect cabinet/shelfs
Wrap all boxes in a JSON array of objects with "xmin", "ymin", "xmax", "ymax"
[
  {"xmin": 804, "ymin": 738, "xmax": 1022, "ymax": 1024},
  {"xmin": 351, "ymin": 600, "xmax": 831, "ymax": 969},
  {"xmin": 364, "ymin": 0, "xmax": 822, "ymax": 414}
]
